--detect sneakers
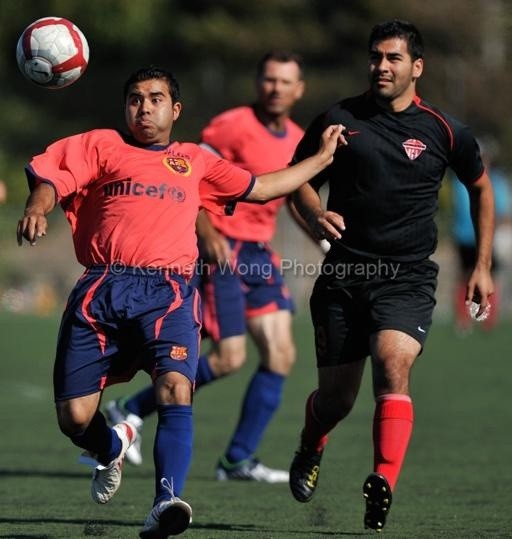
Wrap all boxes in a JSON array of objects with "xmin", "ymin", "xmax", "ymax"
[
  {"xmin": 362, "ymin": 472, "xmax": 392, "ymax": 533},
  {"xmin": 289, "ymin": 426, "xmax": 328, "ymax": 503},
  {"xmin": 215, "ymin": 455, "xmax": 290, "ymax": 484},
  {"xmin": 138, "ymin": 476, "xmax": 193, "ymax": 539},
  {"xmin": 77, "ymin": 421, "xmax": 137, "ymax": 505},
  {"xmin": 104, "ymin": 394, "xmax": 145, "ymax": 467}
]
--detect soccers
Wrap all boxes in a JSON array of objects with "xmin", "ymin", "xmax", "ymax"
[{"xmin": 16, "ymin": 17, "xmax": 90, "ymax": 89}]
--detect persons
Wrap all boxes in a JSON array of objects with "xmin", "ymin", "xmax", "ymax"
[
  {"xmin": 103, "ymin": 45, "xmax": 331, "ymax": 485},
  {"xmin": 285, "ymin": 16, "xmax": 497, "ymax": 535},
  {"xmin": 16, "ymin": 63, "xmax": 353, "ymax": 538},
  {"xmin": 449, "ymin": 141, "xmax": 509, "ymax": 330}
]
